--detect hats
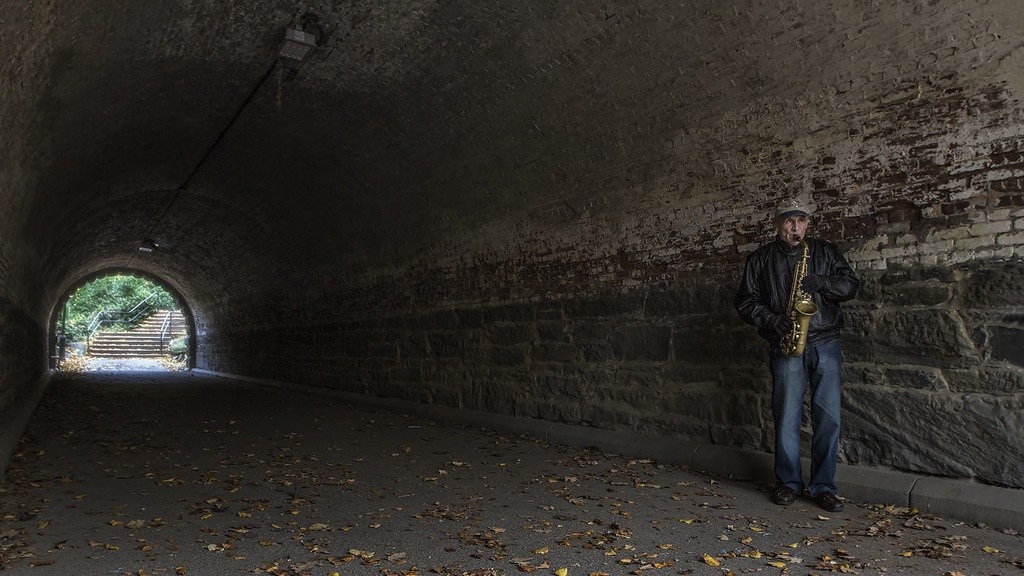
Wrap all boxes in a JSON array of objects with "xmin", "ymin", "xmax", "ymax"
[{"xmin": 775, "ymin": 200, "xmax": 810, "ymax": 217}]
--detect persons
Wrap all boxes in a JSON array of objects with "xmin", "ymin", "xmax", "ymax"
[{"xmin": 735, "ymin": 197, "xmax": 857, "ymax": 511}]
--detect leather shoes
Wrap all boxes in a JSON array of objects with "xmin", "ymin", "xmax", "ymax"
[
  {"xmin": 814, "ymin": 490, "xmax": 844, "ymax": 512},
  {"xmin": 774, "ymin": 486, "xmax": 799, "ymax": 504}
]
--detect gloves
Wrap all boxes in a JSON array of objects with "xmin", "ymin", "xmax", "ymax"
[
  {"xmin": 801, "ymin": 273, "xmax": 825, "ymax": 295},
  {"xmin": 762, "ymin": 313, "xmax": 794, "ymax": 335}
]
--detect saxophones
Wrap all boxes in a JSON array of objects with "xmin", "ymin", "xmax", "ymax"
[{"xmin": 778, "ymin": 234, "xmax": 821, "ymax": 358}]
